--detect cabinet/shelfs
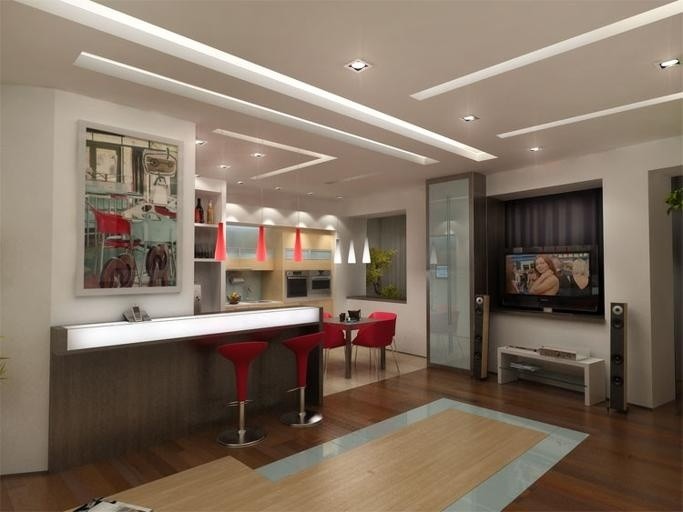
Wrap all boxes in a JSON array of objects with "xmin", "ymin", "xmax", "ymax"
[
  {"xmin": 274, "ymin": 230, "xmax": 335, "ymax": 270},
  {"xmin": 496, "ymin": 345, "xmax": 606, "ymax": 405},
  {"xmin": 193, "ymin": 189, "xmax": 222, "ymax": 262},
  {"xmin": 302, "ymin": 300, "xmax": 333, "ymax": 316}
]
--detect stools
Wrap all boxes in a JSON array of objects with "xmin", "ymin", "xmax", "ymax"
[
  {"xmin": 212, "ymin": 341, "xmax": 273, "ymax": 448},
  {"xmin": 278, "ymin": 328, "xmax": 327, "ymax": 428}
]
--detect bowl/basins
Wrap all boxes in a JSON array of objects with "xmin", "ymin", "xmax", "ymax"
[{"xmin": 227, "ymin": 296, "xmax": 242, "ymax": 304}]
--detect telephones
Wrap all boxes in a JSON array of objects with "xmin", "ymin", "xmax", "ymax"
[{"xmin": 123, "ymin": 304, "xmax": 151, "ymax": 322}]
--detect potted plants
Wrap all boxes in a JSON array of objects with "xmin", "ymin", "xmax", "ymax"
[{"xmin": 365, "ymin": 245, "xmax": 401, "ymax": 300}]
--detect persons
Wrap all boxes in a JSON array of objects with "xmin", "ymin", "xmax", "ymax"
[{"xmin": 505, "ymin": 254, "xmax": 590, "ymax": 296}]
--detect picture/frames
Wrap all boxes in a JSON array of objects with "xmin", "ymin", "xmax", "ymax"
[
  {"xmin": 427, "ymin": 233, "xmax": 457, "ymax": 273},
  {"xmin": 76, "ymin": 118, "xmax": 183, "ymax": 296}
]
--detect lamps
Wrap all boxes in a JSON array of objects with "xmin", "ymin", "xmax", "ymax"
[
  {"xmin": 293, "ymin": 189, "xmax": 302, "ymax": 262},
  {"xmin": 361, "ymin": 206, "xmax": 370, "ymax": 264},
  {"xmin": 256, "ymin": 183, "xmax": 265, "ymax": 261},
  {"xmin": 332, "ymin": 196, "xmax": 342, "ymax": 263},
  {"xmin": 347, "ymin": 206, "xmax": 356, "ymax": 265},
  {"xmin": 211, "ymin": 175, "xmax": 226, "ymax": 262},
  {"xmin": 429, "ymin": 245, "xmax": 437, "ymax": 265}
]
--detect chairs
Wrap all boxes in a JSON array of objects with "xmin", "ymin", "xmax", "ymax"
[
  {"xmin": 429, "ymin": 304, "xmax": 465, "ymax": 363},
  {"xmin": 321, "ymin": 309, "xmax": 401, "ymax": 384},
  {"xmin": 88, "ymin": 201, "xmax": 142, "ymax": 287}
]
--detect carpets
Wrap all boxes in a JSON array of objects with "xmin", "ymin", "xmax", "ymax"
[{"xmin": 318, "ymin": 339, "xmax": 428, "ymax": 398}]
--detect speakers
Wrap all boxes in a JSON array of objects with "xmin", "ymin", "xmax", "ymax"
[
  {"xmin": 608, "ymin": 302, "xmax": 628, "ymax": 411},
  {"xmin": 474, "ymin": 295, "xmax": 490, "ymax": 380}
]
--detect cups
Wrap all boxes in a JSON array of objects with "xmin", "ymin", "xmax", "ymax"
[{"xmin": 339, "ymin": 312, "xmax": 346, "ymax": 322}]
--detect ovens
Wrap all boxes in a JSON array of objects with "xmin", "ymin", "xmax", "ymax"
[{"xmin": 285, "ymin": 269, "xmax": 332, "ymax": 299}]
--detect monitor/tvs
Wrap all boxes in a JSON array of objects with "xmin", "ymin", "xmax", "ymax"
[{"xmin": 496, "ymin": 244, "xmax": 599, "ymax": 313}]
